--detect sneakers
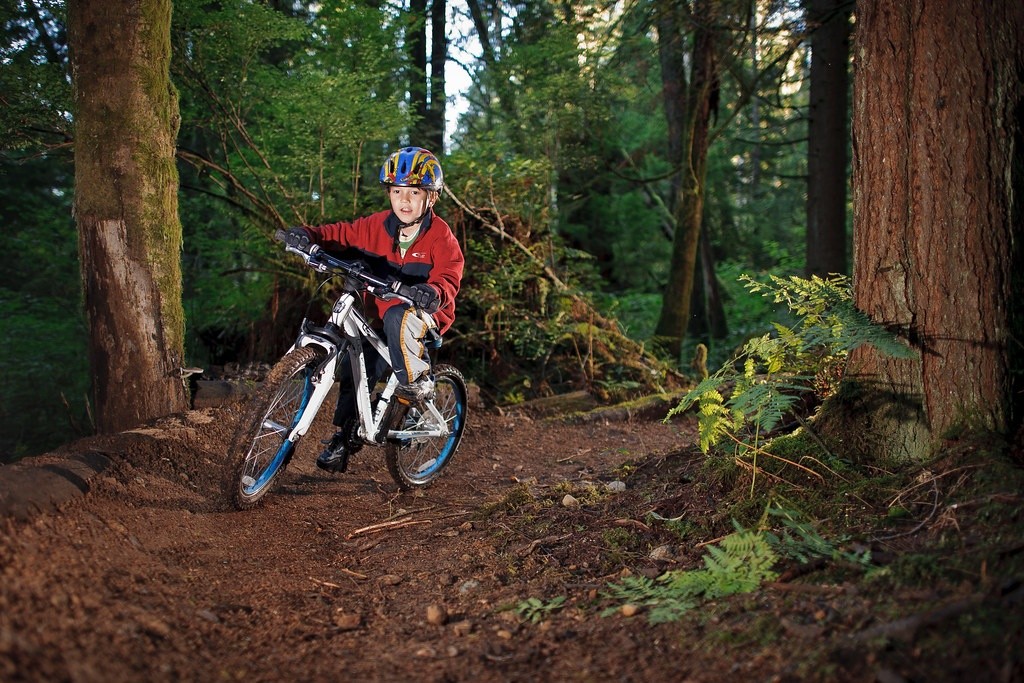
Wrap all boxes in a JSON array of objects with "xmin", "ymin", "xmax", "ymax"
[
  {"xmin": 316, "ymin": 434, "xmax": 350, "ymax": 469},
  {"xmin": 395, "ymin": 375, "xmax": 434, "ymax": 400}
]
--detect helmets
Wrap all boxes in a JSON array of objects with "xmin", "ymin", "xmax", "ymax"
[{"xmin": 380, "ymin": 146, "xmax": 444, "ymax": 195}]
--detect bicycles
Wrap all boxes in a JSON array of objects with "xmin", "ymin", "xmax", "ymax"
[{"xmin": 229, "ymin": 229, "xmax": 469, "ymax": 512}]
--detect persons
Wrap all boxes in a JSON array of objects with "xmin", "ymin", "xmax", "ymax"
[{"xmin": 285, "ymin": 146, "xmax": 465, "ymax": 468}]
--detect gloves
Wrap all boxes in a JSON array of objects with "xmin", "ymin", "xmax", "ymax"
[
  {"xmin": 409, "ymin": 283, "xmax": 439, "ymax": 309},
  {"xmin": 286, "ymin": 227, "xmax": 310, "ymax": 251}
]
